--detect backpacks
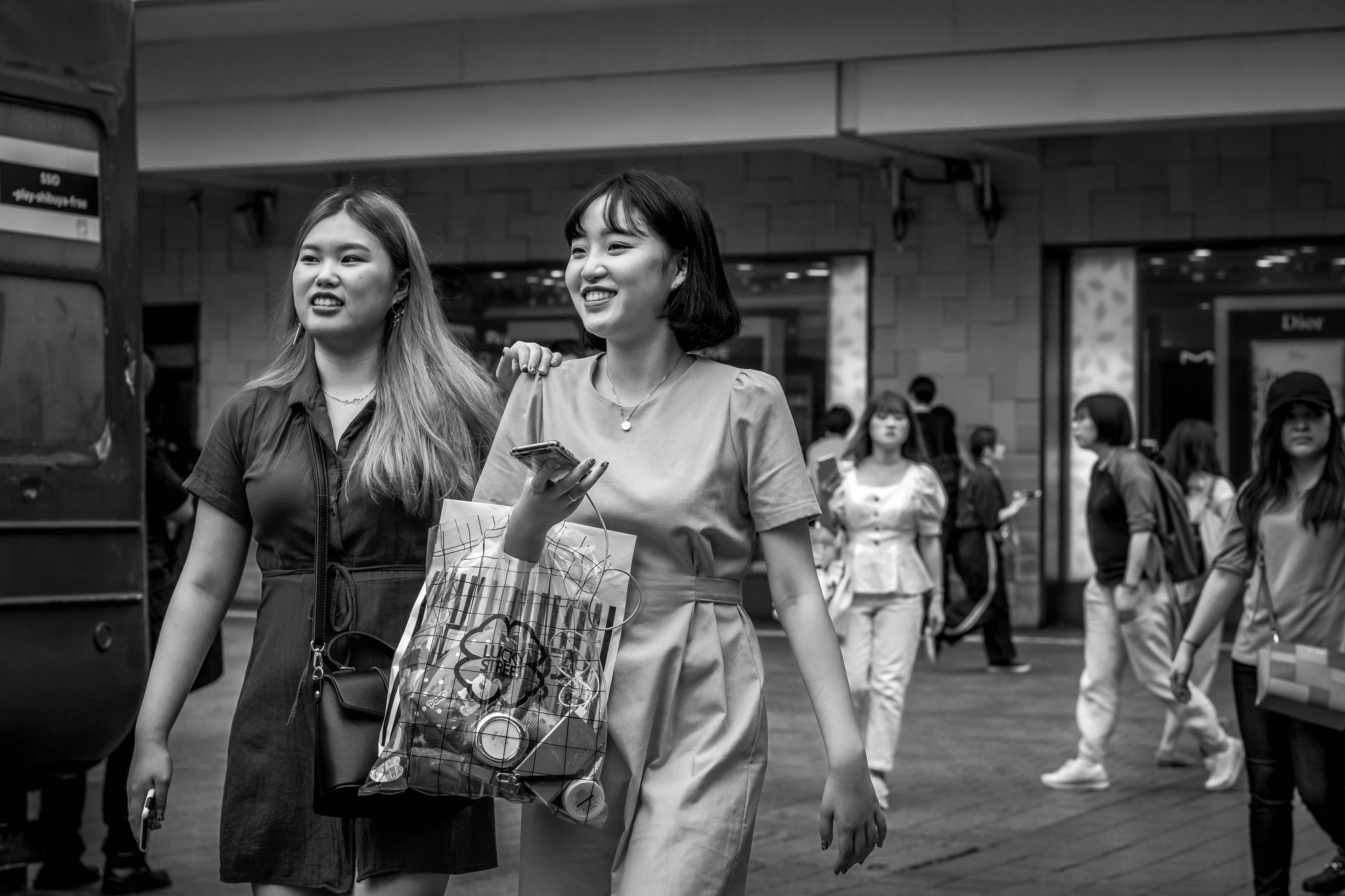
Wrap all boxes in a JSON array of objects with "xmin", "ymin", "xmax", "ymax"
[{"xmin": 1113, "ymin": 452, "xmax": 1206, "ymax": 583}]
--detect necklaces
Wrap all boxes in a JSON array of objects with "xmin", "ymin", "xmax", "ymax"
[
  {"xmin": 606, "ymin": 350, "xmax": 684, "ymax": 430},
  {"xmin": 321, "ymin": 386, "xmax": 376, "ymax": 405}
]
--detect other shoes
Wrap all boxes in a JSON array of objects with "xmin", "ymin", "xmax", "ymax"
[
  {"xmin": 1154, "ymin": 748, "xmax": 1197, "ymax": 769},
  {"xmin": 923, "ymin": 626, "xmax": 944, "ymax": 663},
  {"xmin": 1302, "ymin": 855, "xmax": 1345, "ymax": 893},
  {"xmin": 988, "ymin": 659, "xmax": 1030, "ymax": 675}
]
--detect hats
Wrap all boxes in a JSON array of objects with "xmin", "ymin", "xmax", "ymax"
[{"xmin": 1264, "ymin": 369, "xmax": 1335, "ymax": 414}]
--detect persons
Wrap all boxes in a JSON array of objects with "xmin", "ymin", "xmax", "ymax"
[{"xmin": 125, "ymin": 164, "xmax": 1345, "ymax": 896}]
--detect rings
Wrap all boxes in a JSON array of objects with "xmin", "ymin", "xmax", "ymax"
[{"xmin": 568, "ymin": 490, "xmax": 577, "ymax": 502}]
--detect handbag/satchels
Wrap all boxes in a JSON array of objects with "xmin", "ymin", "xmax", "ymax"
[
  {"xmin": 287, "ymin": 635, "xmax": 491, "ymax": 820},
  {"xmin": 1254, "ymin": 642, "xmax": 1345, "ymax": 731},
  {"xmin": 354, "ymin": 494, "xmax": 637, "ymax": 825}
]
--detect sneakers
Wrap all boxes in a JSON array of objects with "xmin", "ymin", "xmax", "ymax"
[
  {"xmin": 1041, "ymin": 758, "xmax": 1110, "ymax": 791},
  {"xmin": 1206, "ymin": 737, "xmax": 1246, "ymax": 792}
]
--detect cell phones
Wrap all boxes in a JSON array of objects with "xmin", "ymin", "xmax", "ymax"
[
  {"xmin": 816, "ymin": 454, "xmax": 841, "ymax": 481},
  {"xmin": 510, "ymin": 441, "xmax": 593, "ymax": 484},
  {"xmin": 139, "ymin": 788, "xmax": 155, "ymax": 852}
]
